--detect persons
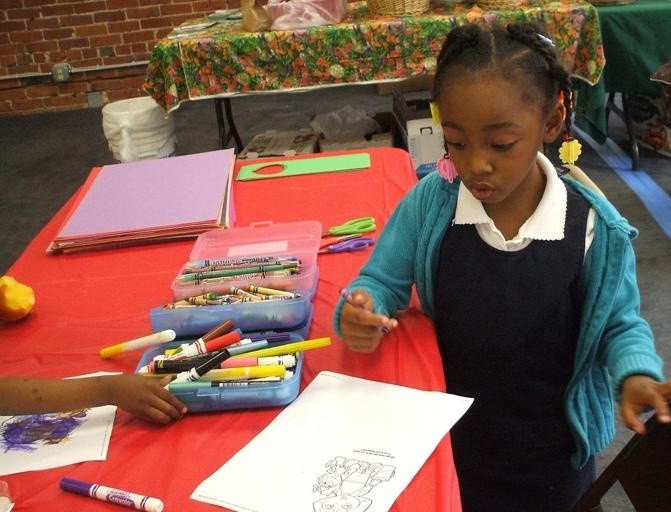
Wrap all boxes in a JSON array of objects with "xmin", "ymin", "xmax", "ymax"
[
  {"xmin": 330, "ymin": 20, "xmax": 670, "ymax": 512},
  {"xmin": 0, "ymin": 370, "xmax": 190, "ymax": 429}
]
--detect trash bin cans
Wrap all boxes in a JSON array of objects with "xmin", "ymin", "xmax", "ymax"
[{"xmin": 101, "ymin": 95, "xmax": 177, "ymax": 164}]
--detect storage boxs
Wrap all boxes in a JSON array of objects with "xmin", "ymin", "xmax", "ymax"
[
  {"xmin": 391, "ymin": 85, "xmax": 447, "ymax": 176},
  {"xmin": 319, "ymin": 111, "xmax": 399, "ymax": 152},
  {"xmin": 621, "ymin": 89, "xmax": 671, "ymax": 158},
  {"xmin": 238, "ymin": 130, "xmax": 320, "ymax": 160}
]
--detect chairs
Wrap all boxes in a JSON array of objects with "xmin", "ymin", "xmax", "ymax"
[{"xmin": 572, "ymin": 400, "xmax": 671, "ymax": 512}]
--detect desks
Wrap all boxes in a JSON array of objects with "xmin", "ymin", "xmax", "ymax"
[
  {"xmin": 141, "ymin": 0, "xmax": 609, "ymax": 157},
  {"xmin": 590, "ymin": 0, "xmax": 670, "ymax": 171},
  {"xmin": 0, "ymin": 146, "xmax": 464, "ymax": 512}
]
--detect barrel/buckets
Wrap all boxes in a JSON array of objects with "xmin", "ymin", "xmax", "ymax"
[{"xmin": 101, "ymin": 95, "xmax": 177, "ymax": 164}]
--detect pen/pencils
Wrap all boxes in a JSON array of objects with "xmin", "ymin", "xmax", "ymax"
[
  {"xmin": 59, "ymin": 477, "xmax": 164, "ymax": 512},
  {"xmin": 99, "ymin": 255, "xmax": 331, "ymax": 414},
  {"xmin": 338, "ymin": 287, "xmax": 390, "ymax": 334}
]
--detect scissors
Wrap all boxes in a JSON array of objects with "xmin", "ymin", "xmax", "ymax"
[{"xmin": 317, "ymin": 216, "xmax": 377, "ymax": 253}]
